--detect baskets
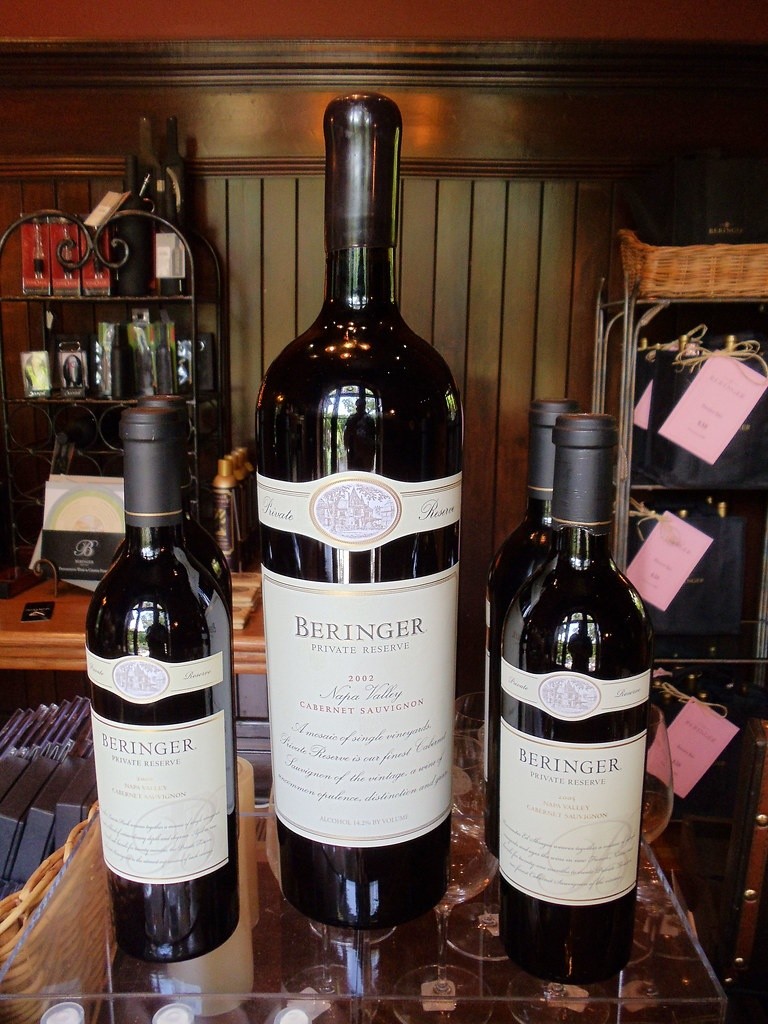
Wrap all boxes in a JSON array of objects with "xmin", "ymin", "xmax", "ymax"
[
  {"xmin": 1, "ymin": 798, "xmax": 121, "ymax": 1021},
  {"xmin": 617, "ymin": 226, "xmax": 768, "ymax": 299}
]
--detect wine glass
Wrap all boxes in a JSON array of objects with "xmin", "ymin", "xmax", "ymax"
[{"xmin": 285, "ymin": 690, "xmax": 673, "ymax": 1024}]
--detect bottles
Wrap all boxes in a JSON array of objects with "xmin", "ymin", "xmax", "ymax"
[
  {"xmin": 501, "ymin": 412, "xmax": 653, "ymax": 982},
  {"xmin": 213, "ymin": 447, "xmax": 256, "ymax": 571},
  {"xmin": 85, "ymin": 395, "xmax": 241, "ymax": 962},
  {"xmin": 654, "ymin": 643, "xmax": 759, "ymax": 838},
  {"xmin": 635, "ymin": 325, "xmax": 765, "ymax": 635},
  {"xmin": 260, "ymin": 94, "xmax": 463, "ymax": 934},
  {"xmin": 484, "ymin": 402, "xmax": 583, "ymax": 857},
  {"xmin": 114, "ymin": 116, "xmax": 189, "ymax": 295}
]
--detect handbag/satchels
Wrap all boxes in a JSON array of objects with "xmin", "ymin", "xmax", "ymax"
[
  {"xmin": 651, "ymin": 664, "xmax": 742, "ymax": 813},
  {"xmin": 628, "ymin": 494, "xmax": 745, "ymax": 637},
  {"xmin": 634, "ymin": 322, "xmax": 768, "ymax": 488}
]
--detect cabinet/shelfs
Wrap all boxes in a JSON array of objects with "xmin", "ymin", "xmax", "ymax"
[
  {"xmin": 597, "ymin": 269, "xmax": 767, "ymax": 830},
  {"xmin": 1, "ymin": 207, "xmax": 237, "ymax": 605}
]
